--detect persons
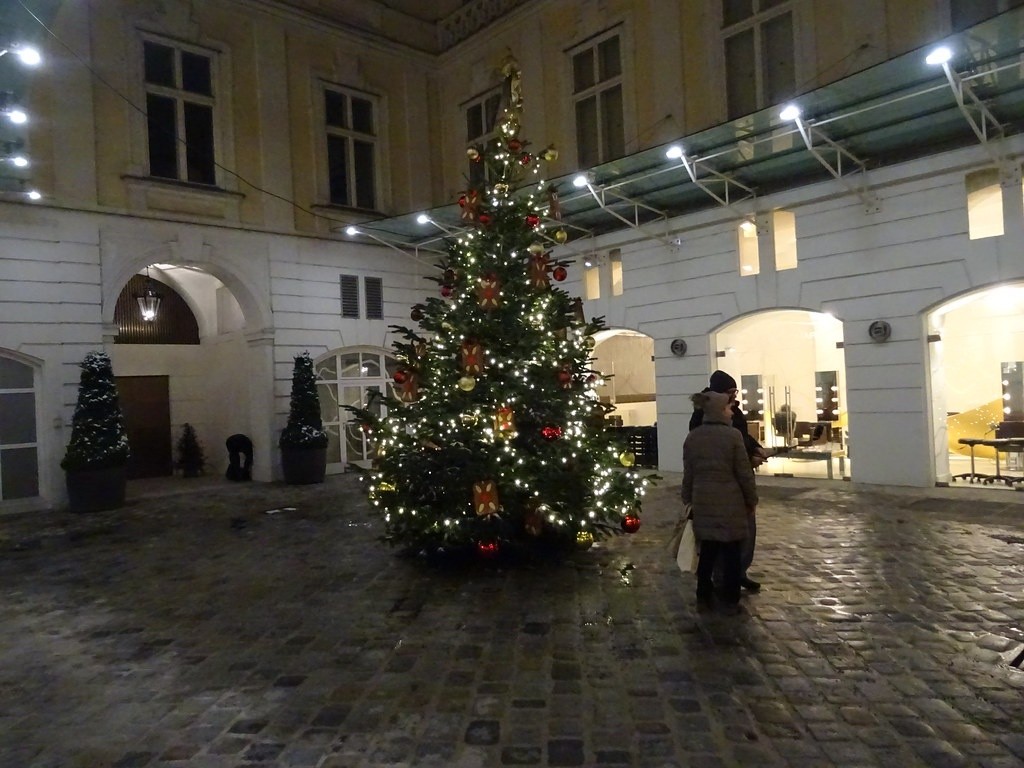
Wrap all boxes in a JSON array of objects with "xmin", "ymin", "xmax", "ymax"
[
  {"xmin": 680, "ymin": 391, "xmax": 758, "ymax": 633},
  {"xmin": 689, "ymin": 370, "xmax": 768, "ymax": 593}
]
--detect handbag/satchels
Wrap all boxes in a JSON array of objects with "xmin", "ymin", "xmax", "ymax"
[{"xmin": 664, "ymin": 502, "xmax": 700, "ymax": 574}]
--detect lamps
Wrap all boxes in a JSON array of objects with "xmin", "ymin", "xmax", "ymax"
[
  {"xmin": 0, "ymin": 39, "xmax": 42, "ymax": 201},
  {"xmin": 927, "ymin": 43, "xmax": 1023, "ymax": 189},
  {"xmin": 781, "ymin": 100, "xmax": 883, "ymax": 215},
  {"xmin": 536, "ymin": 216, "xmax": 609, "ymax": 267},
  {"xmin": 347, "ymin": 227, "xmax": 451, "ymax": 272},
  {"xmin": 574, "ymin": 170, "xmax": 681, "ymax": 254},
  {"xmin": 132, "ymin": 264, "xmax": 164, "ymax": 326},
  {"xmin": 667, "ymin": 142, "xmax": 772, "ymax": 237},
  {"xmin": 417, "ymin": 213, "xmax": 469, "ymax": 241}
]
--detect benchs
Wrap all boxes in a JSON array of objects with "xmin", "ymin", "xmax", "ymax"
[{"xmin": 755, "ymin": 449, "xmax": 845, "ymax": 480}]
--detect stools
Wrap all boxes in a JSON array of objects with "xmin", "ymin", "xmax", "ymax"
[
  {"xmin": 951, "ymin": 439, "xmax": 994, "ymax": 485},
  {"xmin": 1009, "ymin": 437, "xmax": 1024, "ymax": 487},
  {"xmin": 982, "ymin": 438, "xmax": 1022, "ymax": 487}
]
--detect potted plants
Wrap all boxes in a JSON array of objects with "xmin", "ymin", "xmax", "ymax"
[
  {"xmin": 278, "ymin": 350, "xmax": 330, "ymax": 486},
  {"xmin": 173, "ymin": 422, "xmax": 218, "ymax": 478},
  {"xmin": 58, "ymin": 349, "xmax": 132, "ymax": 511}
]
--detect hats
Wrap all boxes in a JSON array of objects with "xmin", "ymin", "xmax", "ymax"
[
  {"xmin": 691, "ymin": 391, "xmax": 730, "ymax": 413},
  {"xmin": 709, "ymin": 370, "xmax": 737, "ymax": 393}
]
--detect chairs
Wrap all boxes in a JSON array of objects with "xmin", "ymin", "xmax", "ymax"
[{"xmin": 796, "ymin": 421, "xmax": 834, "ymax": 450}]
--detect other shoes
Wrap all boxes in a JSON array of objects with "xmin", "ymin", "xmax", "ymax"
[
  {"xmin": 726, "ymin": 585, "xmax": 741, "ymax": 601},
  {"xmin": 740, "ymin": 578, "xmax": 761, "ymax": 591},
  {"xmin": 696, "ymin": 580, "xmax": 714, "ymax": 599}
]
